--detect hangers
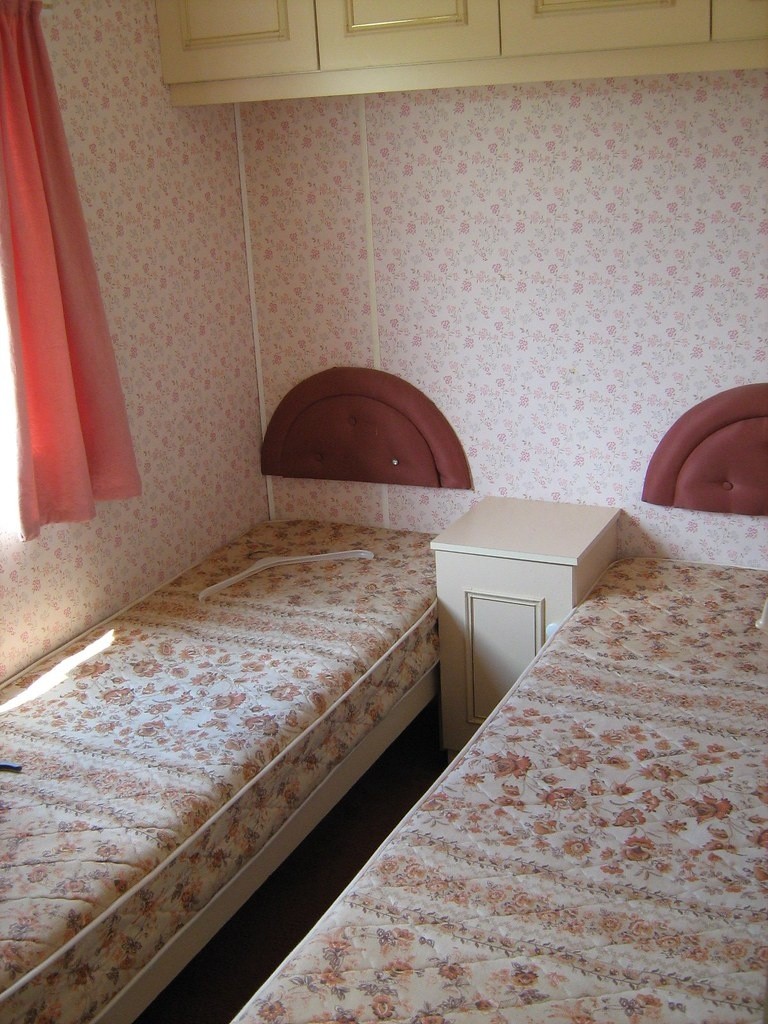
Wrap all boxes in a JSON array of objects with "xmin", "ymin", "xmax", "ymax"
[{"xmin": 198, "ymin": 550, "xmax": 374, "ymax": 605}]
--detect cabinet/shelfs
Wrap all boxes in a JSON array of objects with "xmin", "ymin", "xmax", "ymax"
[
  {"xmin": 499, "ymin": 0, "xmax": 768, "ymax": 85},
  {"xmin": 154, "ymin": 0, "xmax": 502, "ymax": 106}
]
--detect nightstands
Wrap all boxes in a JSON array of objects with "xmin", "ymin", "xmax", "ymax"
[{"xmin": 430, "ymin": 495, "xmax": 621, "ymax": 766}]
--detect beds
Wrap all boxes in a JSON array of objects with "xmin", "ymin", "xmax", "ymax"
[
  {"xmin": 229, "ymin": 383, "xmax": 768, "ymax": 1024},
  {"xmin": 0, "ymin": 366, "xmax": 472, "ymax": 1024}
]
